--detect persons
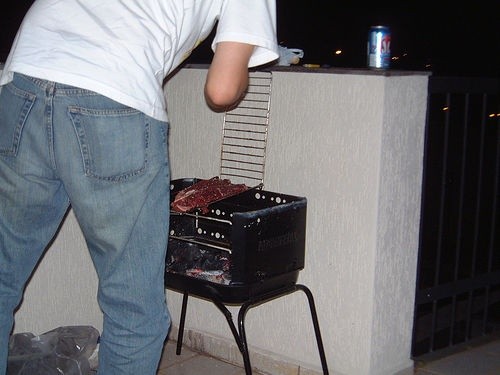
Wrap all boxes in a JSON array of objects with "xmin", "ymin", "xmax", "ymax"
[{"xmin": 0, "ymin": 1, "xmax": 282, "ymax": 374}]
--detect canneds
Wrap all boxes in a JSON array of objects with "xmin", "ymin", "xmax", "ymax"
[{"xmin": 367, "ymin": 25, "xmax": 391, "ymax": 70}]
